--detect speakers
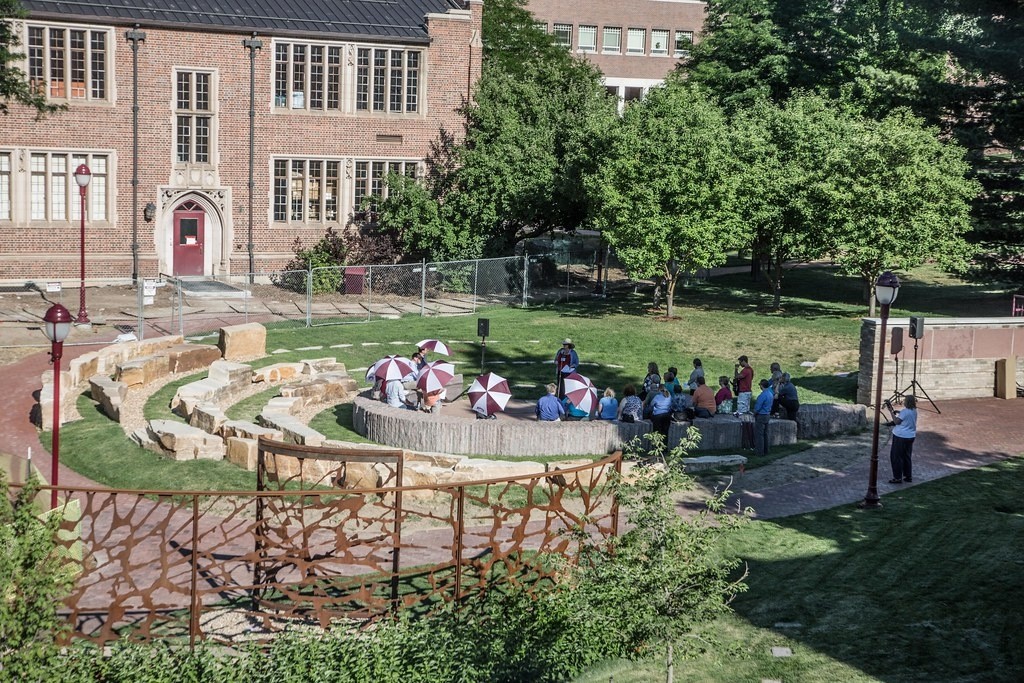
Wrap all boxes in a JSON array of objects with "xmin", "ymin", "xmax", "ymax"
[
  {"xmin": 908, "ymin": 316, "xmax": 924, "ymax": 339},
  {"xmin": 477, "ymin": 318, "xmax": 489, "ymax": 337}
]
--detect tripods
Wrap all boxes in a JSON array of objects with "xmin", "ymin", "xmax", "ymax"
[{"xmin": 882, "ymin": 339, "xmax": 941, "ymax": 415}]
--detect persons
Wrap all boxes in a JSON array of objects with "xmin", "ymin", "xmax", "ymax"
[
  {"xmin": 688, "ymin": 354, "xmax": 799, "ymax": 450},
  {"xmin": 554, "ymin": 338, "xmax": 579, "ymax": 401},
  {"xmin": 619, "ymin": 362, "xmax": 687, "ymax": 452},
  {"xmin": 535, "ymin": 383, "xmax": 564, "ymax": 421},
  {"xmin": 884, "ymin": 395, "xmax": 917, "ymax": 483},
  {"xmin": 599, "ymin": 388, "xmax": 618, "ymax": 420},
  {"xmin": 371, "ymin": 380, "xmax": 416, "ymax": 410},
  {"xmin": 562, "ymin": 396, "xmax": 594, "ymax": 421},
  {"xmin": 412, "ymin": 347, "xmax": 451, "ymax": 406},
  {"xmin": 476, "ymin": 411, "xmax": 497, "ymax": 419}
]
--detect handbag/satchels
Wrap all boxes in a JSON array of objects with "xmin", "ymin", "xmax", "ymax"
[{"xmin": 716, "ymin": 398, "xmax": 733, "ymax": 413}]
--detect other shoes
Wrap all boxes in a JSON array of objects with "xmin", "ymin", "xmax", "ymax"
[
  {"xmin": 903, "ymin": 477, "xmax": 913, "ymax": 482},
  {"xmin": 889, "ymin": 479, "xmax": 902, "ymax": 483}
]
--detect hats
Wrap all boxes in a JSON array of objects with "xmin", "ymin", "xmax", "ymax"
[{"xmin": 562, "ymin": 337, "xmax": 575, "ymax": 349}]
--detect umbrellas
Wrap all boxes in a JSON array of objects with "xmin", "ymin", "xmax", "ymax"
[
  {"xmin": 415, "ymin": 339, "xmax": 452, "ymax": 356},
  {"xmin": 565, "ymin": 374, "xmax": 597, "ymax": 414},
  {"xmin": 417, "ymin": 359, "xmax": 456, "ymax": 393},
  {"xmin": 374, "ymin": 354, "xmax": 418, "ymax": 381},
  {"xmin": 467, "ymin": 372, "xmax": 511, "ymax": 416},
  {"xmin": 366, "ymin": 362, "xmax": 377, "ymax": 382}
]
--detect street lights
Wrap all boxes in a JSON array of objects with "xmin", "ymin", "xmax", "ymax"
[
  {"xmin": 73, "ymin": 163, "xmax": 90, "ymax": 329},
  {"xmin": 40, "ymin": 303, "xmax": 74, "ymax": 507},
  {"xmin": 854, "ymin": 270, "xmax": 902, "ymax": 509}
]
